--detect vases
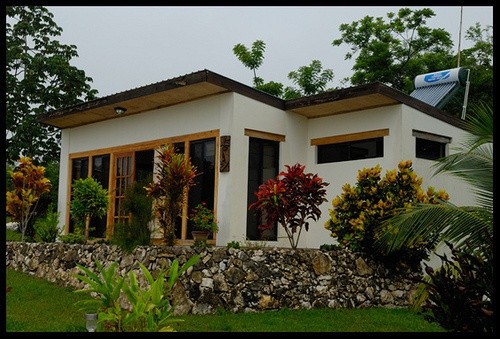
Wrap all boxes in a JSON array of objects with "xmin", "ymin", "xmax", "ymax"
[{"xmin": 193, "ymin": 231, "xmax": 208, "ymax": 246}]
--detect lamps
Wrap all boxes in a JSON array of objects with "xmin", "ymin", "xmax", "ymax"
[{"xmin": 114, "ymin": 107, "xmax": 127, "ymax": 115}]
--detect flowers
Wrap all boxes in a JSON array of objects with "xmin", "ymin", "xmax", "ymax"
[{"xmin": 190, "ymin": 202, "xmax": 220, "ymax": 232}]
